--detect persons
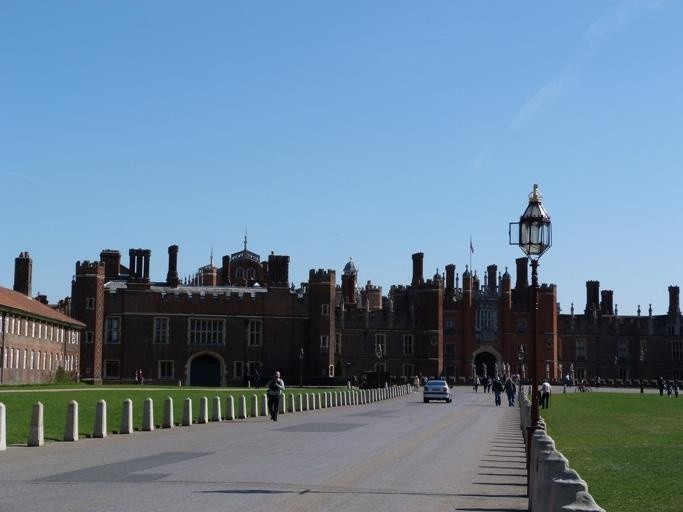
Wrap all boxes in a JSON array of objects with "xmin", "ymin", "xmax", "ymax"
[
  {"xmin": 487, "ymin": 376, "xmax": 493, "ymax": 393},
  {"xmin": 492, "ymin": 376, "xmax": 503, "ymax": 406},
  {"xmin": 266, "ymin": 371, "xmax": 284, "ymax": 421},
  {"xmin": 414, "ymin": 376, "xmax": 419, "ymax": 393},
  {"xmin": 134, "ymin": 368, "xmax": 144, "ymax": 385},
  {"xmin": 503, "ymin": 379, "xmax": 516, "ymax": 407},
  {"xmin": 473, "ymin": 375, "xmax": 481, "ymax": 392},
  {"xmin": 577, "ymin": 376, "xmax": 584, "ymax": 391},
  {"xmin": 482, "ymin": 375, "xmax": 488, "ymax": 393},
  {"xmin": 658, "ymin": 376, "xmax": 679, "ymax": 398},
  {"xmin": 418, "ymin": 375, "xmax": 444, "ymax": 385},
  {"xmin": 596, "ymin": 375, "xmax": 600, "ymax": 387},
  {"xmin": 490, "ymin": 373, "xmax": 521, "ymax": 384},
  {"xmin": 564, "ymin": 371, "xmax": 573, "ymax": 387},
  {"xmin": 540, "ymin": 379, "xmax": 552, "ymax": 408},
  {"xmin": 254, "ymin": 370, "xmax": 260, "ymax": 389}
]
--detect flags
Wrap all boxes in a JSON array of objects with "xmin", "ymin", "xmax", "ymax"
[{"xmin": 470, "ymin": 239, "xmax": 474, "ymax": 254}]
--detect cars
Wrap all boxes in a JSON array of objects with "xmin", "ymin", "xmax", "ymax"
[{"xmin": 424, "ymin": 380, "xmax": 452, "ymax": 403}]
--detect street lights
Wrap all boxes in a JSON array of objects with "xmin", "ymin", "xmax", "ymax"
[{"xmin": 518, "ymin": 185, "xmax": 552, "ymax": 498}]
